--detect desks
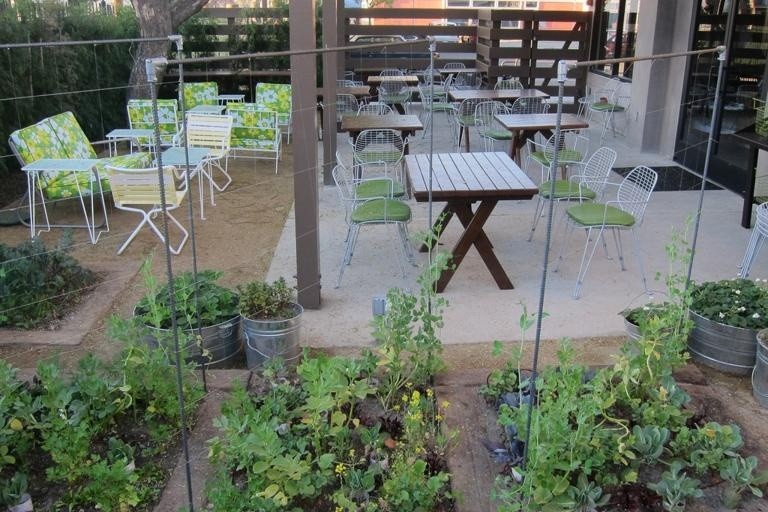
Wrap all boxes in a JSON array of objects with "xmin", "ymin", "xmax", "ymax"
[{"xmin": 402, "ymin": 150, "xmax": 540, "ymax": 293}]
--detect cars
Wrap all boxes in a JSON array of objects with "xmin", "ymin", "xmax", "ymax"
[{"xmin": 343, "ymin": 20, "xmax": 638, "ymax": 84}]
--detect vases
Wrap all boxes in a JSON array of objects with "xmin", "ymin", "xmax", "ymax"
[{"xmin": 750, "ymin": 328, "xmax": 767, "ymax": 408}]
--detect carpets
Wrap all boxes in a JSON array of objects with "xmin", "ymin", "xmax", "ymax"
[{"xmin": 609, "ymin": 165, "xmax": 725, "ymax": 192}]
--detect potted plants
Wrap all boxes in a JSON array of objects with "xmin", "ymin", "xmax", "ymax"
[
  {"xmin": 130, "ymin": 266, "xmax": 304, "ymax": 379},
  {"xmin": 615, "ymin": 276, "xmax": 767, "ymax": 376}
]
